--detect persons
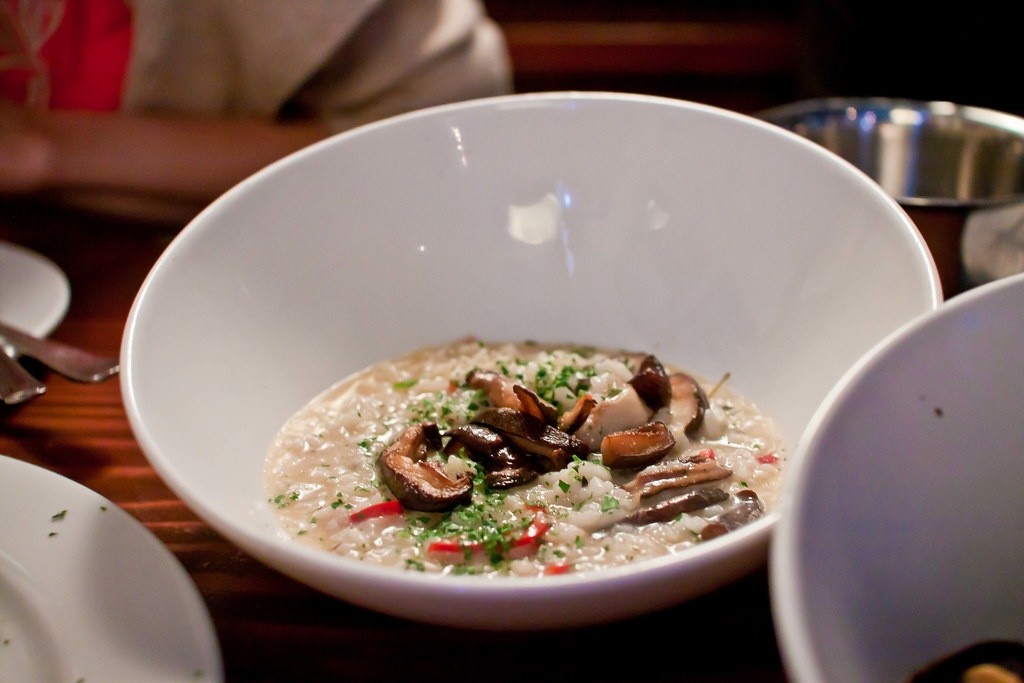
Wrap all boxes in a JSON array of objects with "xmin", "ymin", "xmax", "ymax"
[{"xmin": 0, "ymin": 0, "xmax": 515, "ymax": 220}]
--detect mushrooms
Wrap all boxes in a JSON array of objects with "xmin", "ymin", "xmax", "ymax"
[{"xmin": 378, "ymin": 353, "xmax": 764, "ymax": 540}]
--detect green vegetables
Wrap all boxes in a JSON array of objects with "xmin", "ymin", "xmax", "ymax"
[{"xmin": 270, "ymin": 336, "xmax": 640, "ymax": 575}]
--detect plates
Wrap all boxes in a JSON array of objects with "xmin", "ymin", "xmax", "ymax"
[
  {"xmin": 0, "ymin": 456, "xmax": 224, "ymax": 683},
  {"xmin": 0, "ymin": 240, "xmax": 73, "ymax": 362}
]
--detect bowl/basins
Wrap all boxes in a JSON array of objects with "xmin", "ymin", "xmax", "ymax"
[
  {"xmin": 756, "ymin": 97, "xmax": 1024, "ymax": 301},
  {"xmin": 120, "ymin": 90, "xmax": 945, "ymax": 628},
  {"xmin": 772, "ymin": 274, "xmax": 1024, "ymax": 683}
]
